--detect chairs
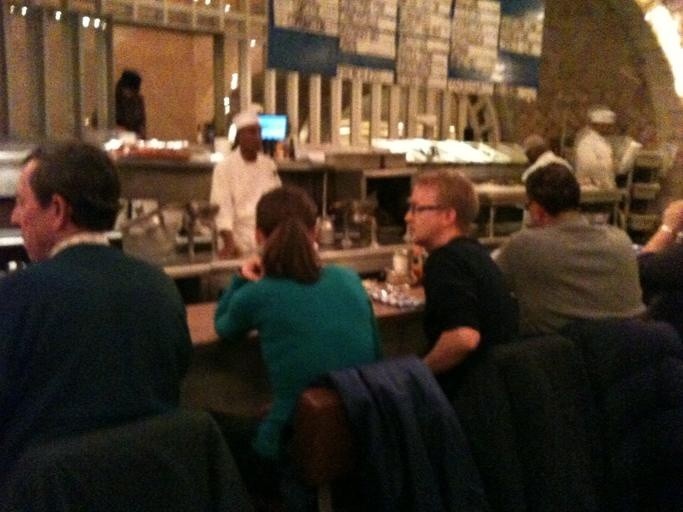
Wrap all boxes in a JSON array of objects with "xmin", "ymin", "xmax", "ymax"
[
  {"xmin": 296, "ymin": 386, "xmax": 361, "ymax": 512},
  {"xmin": 465, "ymin": 319, "xmax": 681, "ymax": 509},
  {"xmin": 18, "ymin": 407, "xmax": 225, "ymax": 512}
]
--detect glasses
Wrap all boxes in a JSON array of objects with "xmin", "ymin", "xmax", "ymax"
[
  {"xmin": 409, "ymin": 203, "xmax": 443, "ymax": 214},
  {"xmin": 524, "ymin": 200, "xmax": 533, "ymax": 211}
]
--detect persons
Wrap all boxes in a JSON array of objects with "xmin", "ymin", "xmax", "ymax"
[
  {"xmin": 522, "ymin": 135, "xmax": 578, "ymax": 232},
  {"xmin": 401, "ymin": 169, "xmax": 520, "ymax": 409},
  {"xmin": 215, "ymin": 182, "xmax": 381, "ymax": 511},
  {"xmin": 636, "ymin": 196, "xmax": 682, "ymax": 338},
  {"xmin": 573, "ymin": 106, "xmax": 623, "ymax": 228},
  {"xmin": 89, "ymin": 69, "xmax": 151, "ymax": 141},
  {"xmin": 1, "ymin": 136, "xmax": 197, "ymax": 480},
  {"xmin": 208, "ymin": 107, "xmax": 284, "ymax": 261},
  {"xmin": 487, "ymin": 160, "xmax": 649, "ymax": 344}
]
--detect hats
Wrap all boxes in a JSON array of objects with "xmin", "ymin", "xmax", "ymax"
[
  {"xmin": 233, "ymin": 110, "xmax": 259, "ymax": 130},
  {"xmin": 589, "ymin": 109, "xmax": 616, "ymax": 123}
]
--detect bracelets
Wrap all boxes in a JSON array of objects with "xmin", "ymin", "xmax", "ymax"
[{"xmin": 659, "ymin": 223, "xmax": 682, "ymax": 239}]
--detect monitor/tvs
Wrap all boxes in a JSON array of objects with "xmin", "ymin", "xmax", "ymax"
[{"xmin": 258, "ymin": 113, "xmax": 290, "ymax": 143}]
